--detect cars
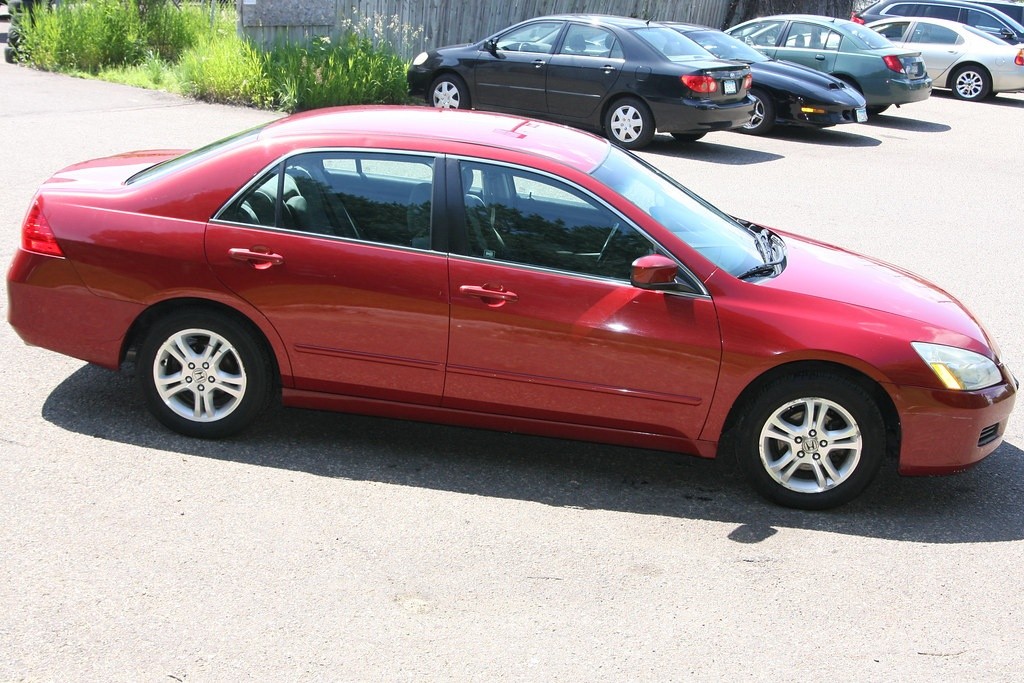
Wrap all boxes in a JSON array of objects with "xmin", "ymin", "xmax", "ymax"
[
  {"xmin": 784, "ymin": 15, "xmax": 1023, "ymax": 101},
  {"xmin": 7, "ymin": 102, "xmax": 1020, "ymax": 511},
  {"xmin": 404, "ymin": 12, "xmax": 757, "ymax": 148},
  {"xmin": 723, "ymin": 14, "xmax": 932, "ymax": 116}
]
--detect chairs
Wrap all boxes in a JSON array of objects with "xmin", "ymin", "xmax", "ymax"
[
  {"xmin": 600, "ymin": 34, "xmax": 621, "ymax": 58},
  {"xmin": 566, "ymin": 34, "xmax": 588, "ymax": 55},
  {"xmin": 406, "ymin": 182, "xmax": 431, "ymax": 251},
  {"xmin": 461, "ymin": 166, "xmax": 507, "ymax": 259}
]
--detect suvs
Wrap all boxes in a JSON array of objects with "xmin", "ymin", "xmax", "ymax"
[{"xmin": 850, "ymin": 0, "xmax": 1024, "ymax": 43}]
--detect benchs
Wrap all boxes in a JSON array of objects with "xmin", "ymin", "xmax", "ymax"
[{"xmin": 229, "ymin": 159, "xmax": 370, "ymax": 241}]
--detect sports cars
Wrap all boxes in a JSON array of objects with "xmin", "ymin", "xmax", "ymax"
[{"xmin": 585, "ymin": 19, "xmax": 868, "ymax": 136}]
